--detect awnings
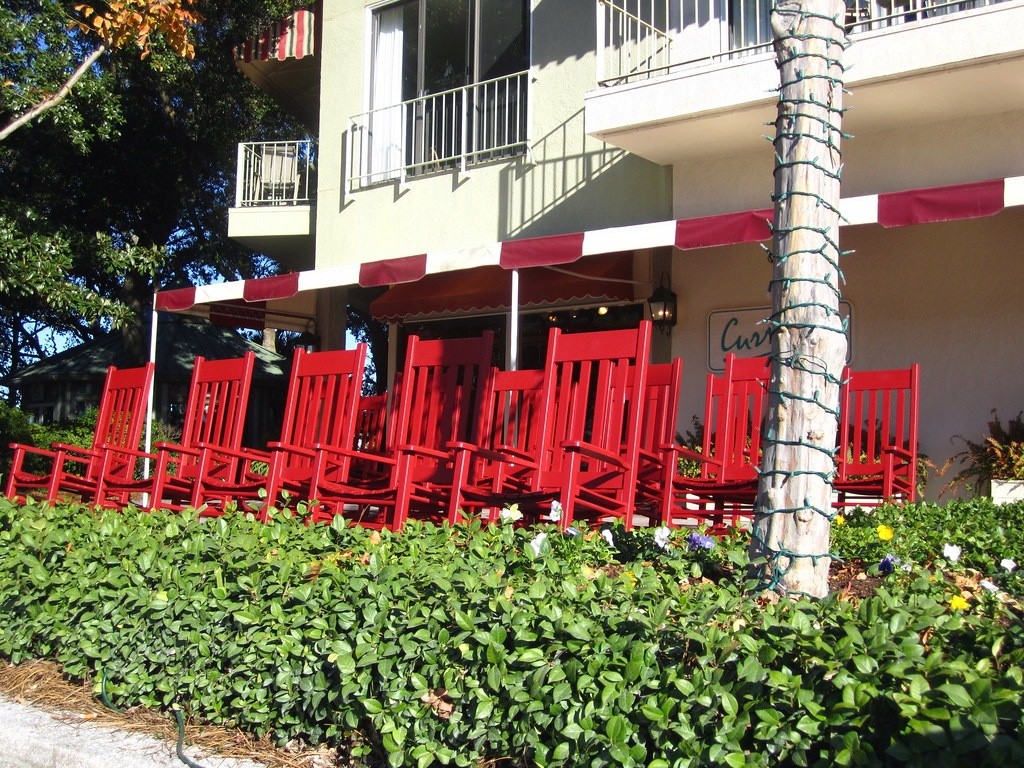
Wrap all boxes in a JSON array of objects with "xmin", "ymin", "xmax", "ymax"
[
  {"xmin": 232, "ymin": 0, "xmax": 323, "ymax": 136},
  {"xmin": 370, "ymin": 251, "xmax": 635, "ymax": 326}
]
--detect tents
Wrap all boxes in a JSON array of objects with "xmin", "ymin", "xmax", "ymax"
[{"xmin": 142, "ymin": 177, "xmax": 1024, "ymax": 510}]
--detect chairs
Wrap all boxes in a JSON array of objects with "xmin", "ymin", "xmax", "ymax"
[
  {"xmin": 488, "ymin": 319, "xmax": 653, "ymax": 529},
  {"xmin": 662, "ymin": 353, "xmax": 772, "ymax": 527},
  {"xmin": 460, "ymin": 368, "xmax": 572, "ymax": 520},
  {"xmin": 305, "ymin": 329, "xmax": 496, "ymax": 530},
  {"xmin": 832, "ymin": 363, "xmax": 920, "ymax": 515},
  {"xmin": 593, "ymin": 357, "xmax": 681, "ymax": 529},
  {"xmin": 387, "ymin": 371, "xmax": 407, "ymax": 461},
  {"xmin": 5, "ymin": 362, "xmax": 156, "ymax": 505},
  {"xmin": 665, "ymin": 375, "xmax": 769, "ymax": 528},
  {"xmin": 188, "ymin": 342, "xmax": 366, "ymax": 522},
  {"xmin": 348, "ymin": 390, "xmax": 390, "ymax": 515},
  {"xmin": 94, "ymin": 349, "xmax": 255, "ymax": 514}
]
